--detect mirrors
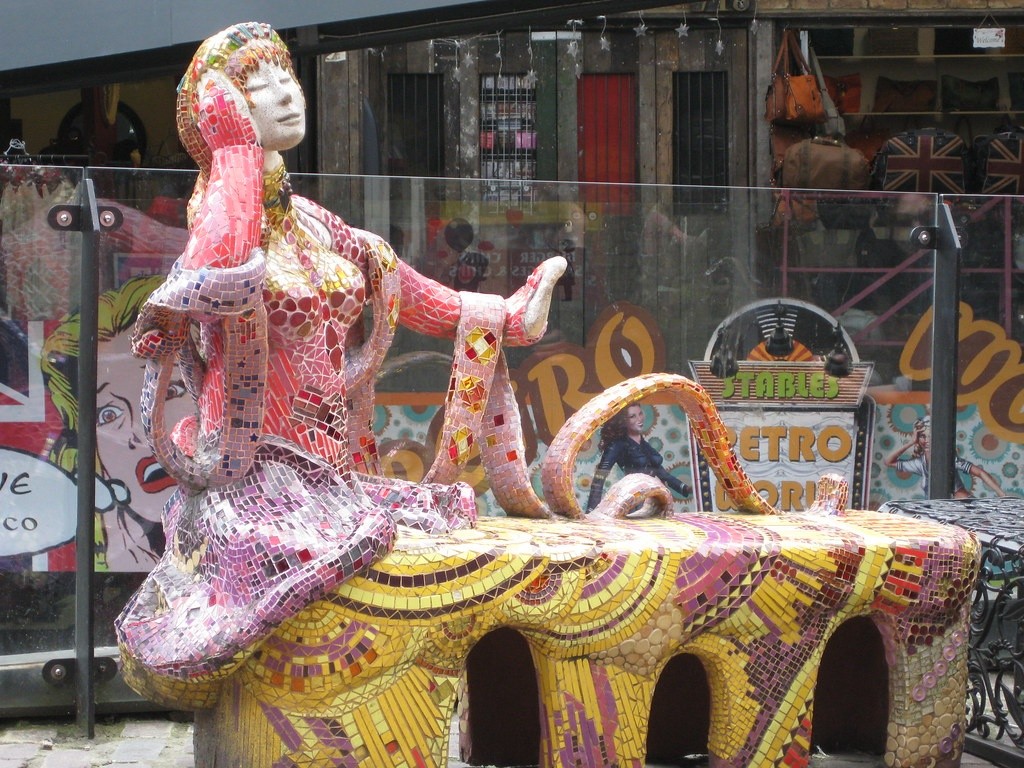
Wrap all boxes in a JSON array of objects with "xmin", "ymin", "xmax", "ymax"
[{"xmin": 57, "ymin": 101, "xmax": 148, "ymax": 190}]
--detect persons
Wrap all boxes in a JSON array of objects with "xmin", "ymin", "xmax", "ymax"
[
  {"xmin": 642, "ymin": 189, "xmax": 711, "ymax": 287},
  {"xmin": 112, "ymin": 24, "xmax": 566, "ymax": 700}
]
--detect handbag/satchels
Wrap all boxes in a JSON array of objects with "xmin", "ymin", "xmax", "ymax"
[{"xmin": 756, "ymin": 29, "xmax": 1024, "ymax": 285}]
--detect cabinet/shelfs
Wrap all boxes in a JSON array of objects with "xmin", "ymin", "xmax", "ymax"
[{"xmin": 816, "ymin": 53, "xmax": 1024, "ymax": 116}]
